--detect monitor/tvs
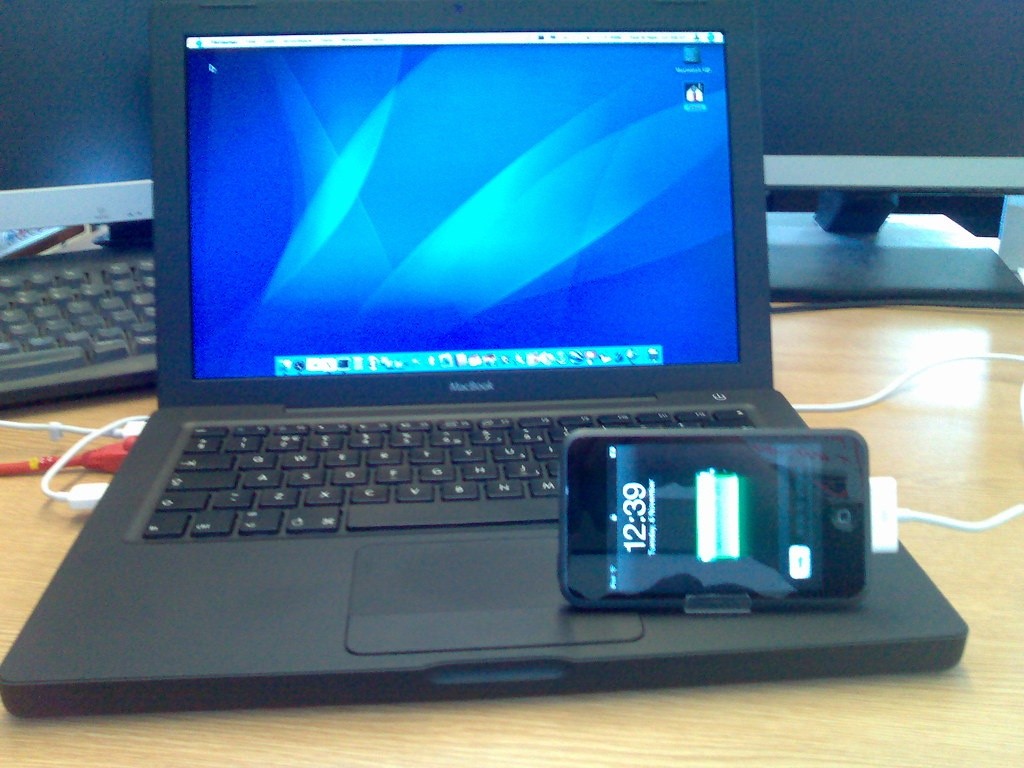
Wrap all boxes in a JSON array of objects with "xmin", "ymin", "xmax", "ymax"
[
  {"xmin": 1, "ymin": 0, "xmax": 153, "ymax": 245},
  {"xmin": 754, "ymin": 0, "xmax": 1024, "ymax": 311}
]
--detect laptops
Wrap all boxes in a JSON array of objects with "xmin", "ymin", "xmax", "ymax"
[{"xmin": 0, "ymin": 0, "xmax": 968, "ymax": 717}]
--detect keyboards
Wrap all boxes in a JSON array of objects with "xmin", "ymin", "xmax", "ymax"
[{"xmin": 0, "ymin": 241, "xmax": 155, "ymax": 410}]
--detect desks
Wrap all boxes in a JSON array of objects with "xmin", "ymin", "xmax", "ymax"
[{"xmin": 0, "ymin": 214, "xmax": 1024, "ymax": 768}]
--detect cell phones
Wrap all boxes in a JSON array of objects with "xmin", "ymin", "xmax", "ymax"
[{"xmin": 555, "ymin": 428, "xmax": 870, "ymax": 609}]
